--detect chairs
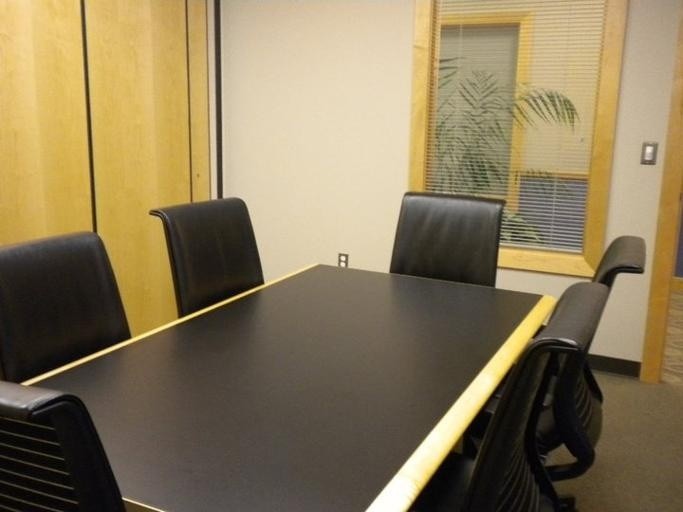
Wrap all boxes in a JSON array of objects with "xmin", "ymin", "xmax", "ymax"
[
  {"xmin": 407, "ymin": 281, "xmax": 609, "ymax": 512},
  {"xmin": 388, "ymin": 192, "xmax": 506, "ymax": 288},
  {"xmin": 149, "ymin": 197, "xmax": 265, "ymax": 319},
  {"xmin": 461, "ymin": 235, "xmax": 646, "ymax": 483},
  {"xmin": 0, "ymin": 231, "xmax": 132, "ymax": 385},
  {"xmin": 0, "ymin": 379, "xmax": 127, "ymax": 511}
]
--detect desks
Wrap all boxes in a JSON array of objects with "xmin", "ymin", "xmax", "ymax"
[{"xmin": 18, "ymin": 263, "xmax": 560, "ymax": 511}]
